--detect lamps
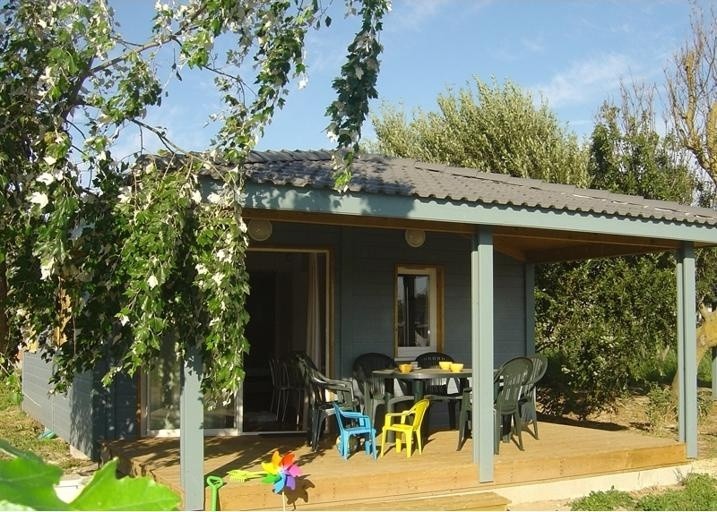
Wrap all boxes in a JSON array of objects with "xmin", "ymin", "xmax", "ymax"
[
  {"xmin": 404, "ymin": 229, "xmax": 425, "ymax": 248},
  {"xmin": 246, "ymin": 220, "xmax": 273, "ymax": 245}
]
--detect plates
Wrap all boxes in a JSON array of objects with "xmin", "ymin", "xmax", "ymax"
[{"xmin": 413, "ymin": 367, "xmax": 421, "ymax": 370}]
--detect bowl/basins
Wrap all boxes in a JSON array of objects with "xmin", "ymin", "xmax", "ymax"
[
  {"xmin": 438, "ymin": 361, "xmax": 451, "ymax": 370},
  {"xmin": 398, "ymin": 364, "xmax": 412, "ymax": 374},
  {"xmin": 450, "ymin": 364, "xmax": 463, "ymax": 372}
]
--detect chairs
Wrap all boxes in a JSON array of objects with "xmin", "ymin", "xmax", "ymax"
[{"xmin": 263, "ymin": 346, "xmax": 549, "ymax": 460}]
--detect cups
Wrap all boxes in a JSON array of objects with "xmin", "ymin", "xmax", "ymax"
[{"xmin": 409, "ymin": 361, "xmax": 417, "ymax": 368}]
